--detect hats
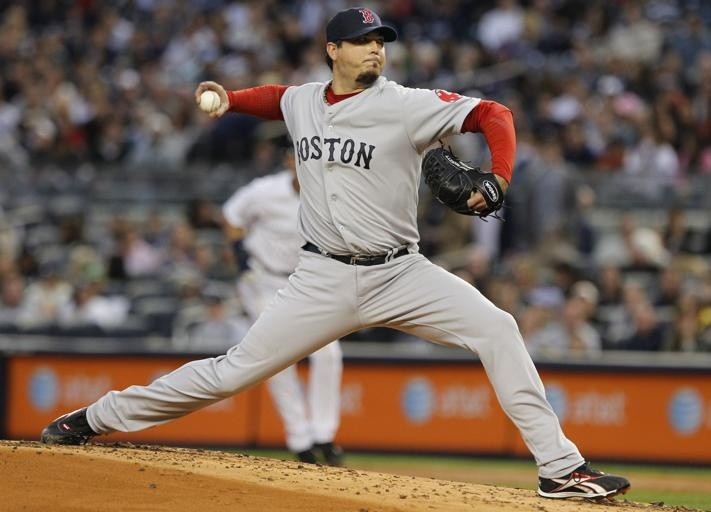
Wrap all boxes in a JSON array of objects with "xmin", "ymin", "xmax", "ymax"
[
  {"xmin": 569, "ymin": 279, "xmax": 599, "ymax": 305},
  {"xmin": 326, "ymin": 6, "xmax": 398, "ymax": 42},
  {"xmin": 631, "ymin": 229, "xmax": 669, "ymax": 267}
]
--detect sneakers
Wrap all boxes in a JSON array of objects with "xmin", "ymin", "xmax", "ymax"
[
  {"xmin": 538, "ymin": 461, "xmax": 631, "ymax": 501},
  {"xmin": 316, "ymin": 443, "xmax": 343, "ymax": 466},
  {"xmin": 40, "ymin": 408, "xmax": 101, "ymax": 448},
  {"xmin": 297, "ymin": 450, "xmax": 315, "ymax": 465}
]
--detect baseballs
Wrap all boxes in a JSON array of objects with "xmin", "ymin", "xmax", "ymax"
[{"xmin": 199, "ymin": 90, "xmax": 221, "ymax": 112}]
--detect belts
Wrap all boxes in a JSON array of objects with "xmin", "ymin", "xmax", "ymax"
[{"xmin": 300, "ymin": 242, "xmax": 412, "ymax": 266}]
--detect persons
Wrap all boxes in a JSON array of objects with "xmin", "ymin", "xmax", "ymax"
[
  {"xmin": 216, "ymin": 139, "xmax": 348, "ymax": 468},
  {"xmin": 1, "ymin": 1, "xmax": 711, "ymax": 353},
  {"xmin": 40, "ymin": 5, "xmax": 635, "ymax": 501}
]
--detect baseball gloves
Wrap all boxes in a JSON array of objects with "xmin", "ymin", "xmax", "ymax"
[{"xmin": 420, "ymin": 148, "xmax": 504, "ymax": 216}]
